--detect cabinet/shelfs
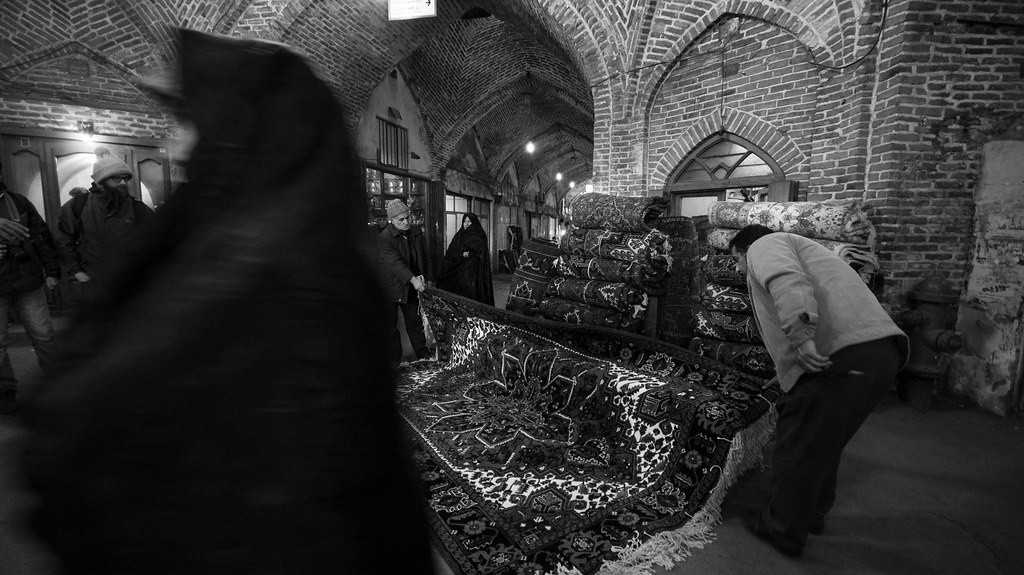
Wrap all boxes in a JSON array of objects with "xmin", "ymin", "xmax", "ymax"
[{"xmin": 367, "ymin": 177, "xmax": 425, "ymax": 215}]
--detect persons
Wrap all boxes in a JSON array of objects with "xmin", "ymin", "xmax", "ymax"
[
  {"xmin": 0, "ymin": 162, "xmax": 62, "ymax": 412},
  {"xmin": 57, "ymin": 149, "xmax": 154, "ymax": 309},
  {"xmin": 729, "ymin": 224, "xmax": 910, "ymax": 559},
  {"xmin": 443, "ymin": 213, "xmax": 495, "ymax": 307},
  {"xmin": 369, "ymin": 198, "xmax": 433, "ymax": 367}
]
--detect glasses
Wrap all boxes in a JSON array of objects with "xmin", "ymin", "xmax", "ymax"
[{"xmin": 110, "ymin": 176, "xmax": 130, "ymax": 183}]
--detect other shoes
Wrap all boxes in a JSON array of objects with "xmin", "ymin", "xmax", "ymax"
[
  {"xmin": 0, "ymin": 391, "xmax": 16, "ymax": 415},
  {"xmin": 808, "ymin": 524, "xmax": 822, "ymax": 536},
  {"xmin": 744, "ymin": 511, "xmax": 801, "ymax": 558}
]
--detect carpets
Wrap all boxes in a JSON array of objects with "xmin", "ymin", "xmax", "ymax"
[{"xmin": 390, "ymin": 283, "xmax": 781, "ymax": 575}]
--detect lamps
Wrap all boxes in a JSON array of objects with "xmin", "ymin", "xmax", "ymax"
[{"xmin": 77, "ymin": 121, "xmax": 95, "ymax": 137}]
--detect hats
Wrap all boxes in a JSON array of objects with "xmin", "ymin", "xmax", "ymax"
[
  {"xmin": 91, "ymin": 149, "xmax": 134, "ymax": 185},
  {"xmin": 385, "ymin": 199, "xmax": 409, "ymax": 219}
]
